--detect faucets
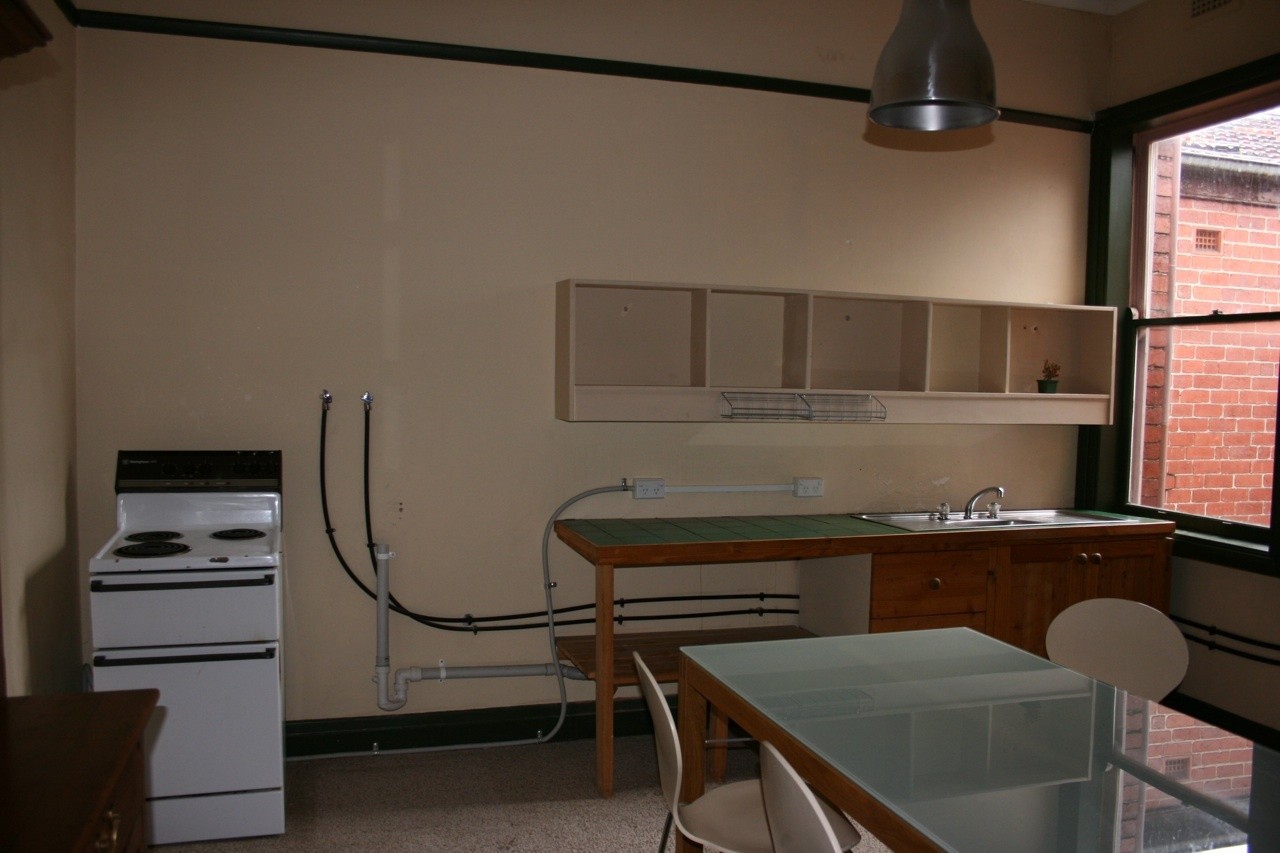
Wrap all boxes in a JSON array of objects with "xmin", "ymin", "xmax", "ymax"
[
  {"xmin": 963, "ymin": 486, "xmax": 1004, "ymax": 520},
  {"xmin": 929, "ymin": 503, "xmax": 950, "ymax": 521}
]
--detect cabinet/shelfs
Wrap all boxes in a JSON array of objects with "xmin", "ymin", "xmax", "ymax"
[
  {"xmin": 553, "ymin": 513, "xmax": 915, "ymax": 799},
  {"xmin": 798, "ymin": 543, "xmax": 1000, "ymax": 637},
  {"xmin": 1002, "ymin": 534, "xmax": 1173, "ymax": 661},
  {"xmin": 0, "ymin": 688, "xmax": 160, "ymax": 853},
  {"xmin": 554, "ymin": 280, "xmax": 1118, "ymax": 425},
  {"xmin": 789, "ymin": 692, "xmax": 1096, "ymax": 802}
]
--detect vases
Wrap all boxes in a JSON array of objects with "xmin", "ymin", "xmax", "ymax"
[{"xmin": 1037, "ymin": 380, "xmax": 1059, "ymax": 393}]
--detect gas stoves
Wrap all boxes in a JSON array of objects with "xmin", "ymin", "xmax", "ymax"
[{"xmin": 86, "ymin": 448, "xmax": 284, "ymax": 572}]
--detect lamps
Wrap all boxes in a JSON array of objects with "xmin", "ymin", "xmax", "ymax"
[{"xmin": 867, "ymin": 0, "xmax": 1001, "ymax": 133}]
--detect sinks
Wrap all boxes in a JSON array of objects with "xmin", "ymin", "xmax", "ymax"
[{"xmin": 943, "ymin": 518, "xmax": 1048, "ymax": 528}]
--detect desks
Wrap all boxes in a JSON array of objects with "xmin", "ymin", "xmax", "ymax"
[{"xmin": 678, "ymin": 626, "xmax": 1280, "ymax": 853}]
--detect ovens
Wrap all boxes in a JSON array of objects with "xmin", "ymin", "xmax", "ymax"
[{"xmin": 89, "ymin": 570, "xmax": 286, "ymax": 844}]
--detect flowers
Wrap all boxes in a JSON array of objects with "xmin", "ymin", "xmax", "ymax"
[{"xmin": 1042, "ymin": 360, "xmax": 1061, "ymax": 380}]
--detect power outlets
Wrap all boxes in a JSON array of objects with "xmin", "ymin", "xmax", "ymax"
[
  {"xmin": 631, "ymin": 478, "xmax": 664, "ymax": 499},
  {"xmin": 793, "ymin": 477, "xmax": 824, "ymax": 497}
]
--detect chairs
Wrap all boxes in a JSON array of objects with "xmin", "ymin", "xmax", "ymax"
[
  {"xmin": 760, "ymin": 739, "xmax": 861, "ymax": 853},
  {"xmin": 633, "ymin": 651, "xmax": 775, "ymax": 853},
  {"xmin": 1045, "ymin": 598, "xmax": 1188, "ymax": 703}
]
